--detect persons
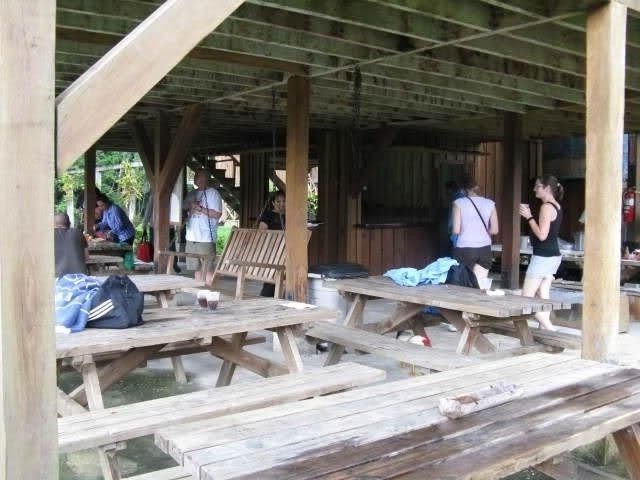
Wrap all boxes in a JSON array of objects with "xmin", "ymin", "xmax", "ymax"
[
  {"xmin": 450, "ymin": 173, "xmax": 500, "ymax": 289},
  {"xmin": 259, "ymin": 191, "xmax": 286, "ymax": 297},
  {"xmin": 519, "ymin": 172, "xmax": 569, "ymax": 353},
  {"xmin": 55, "ymin": 211, "xmax": 89, "ymax": 278},
  {"xmin": 141, "ymin": 193, "xmax": 182, "ymax": 273},
  {"xmin": 184, "ymin": 168, "xmax": 223, "ymax": 289},
  {"xmin": 443, "ymin": 179, "xmax": 464, "ymax": 248},
  {"xmin": 93, "ymin": 193, "xmax": 137, "ymax": 246}
]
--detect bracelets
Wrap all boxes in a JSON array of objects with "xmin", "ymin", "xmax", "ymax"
[{"xmin": 527, "ymin": 216, "xmax": 534, "ymax": 222}]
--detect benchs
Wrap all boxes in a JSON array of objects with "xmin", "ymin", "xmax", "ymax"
[{"xmin": 158, "ymin": 224, "xmax": 312, "ymax": 300}]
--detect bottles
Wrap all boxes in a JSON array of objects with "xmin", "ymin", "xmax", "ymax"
[{"xmin": 624, "ymin": 245, "xmax": 640, "ymax": 260}]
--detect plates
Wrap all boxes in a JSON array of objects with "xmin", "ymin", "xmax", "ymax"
[
  {"xmin": 560, "ymin": 242, "xmax": 586, "ymax": 256},
  {"xmin": 92, "ymin": 239, "xmax": 104, "ymax": 243}
]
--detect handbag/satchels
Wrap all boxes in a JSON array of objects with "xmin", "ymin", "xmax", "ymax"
[
  {"xmin": 86, "ymin": 271, "xmax": 147, "ymax": 330},
  {"xmin": 134, "ymin": 235, "xmax": 154, "ymax": 264}
]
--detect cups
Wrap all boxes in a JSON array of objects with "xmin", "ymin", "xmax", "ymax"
[
  {"xmin": 519, "ymin": 203, "xmax": 529, "ymax": 208},
  {"xmin": 196, "ymin": 288, "xmax": 220, "ymax": 309},
  {"xmin": 521, "ymin": 235, "xmax": 529, "ymax": 249}
]
